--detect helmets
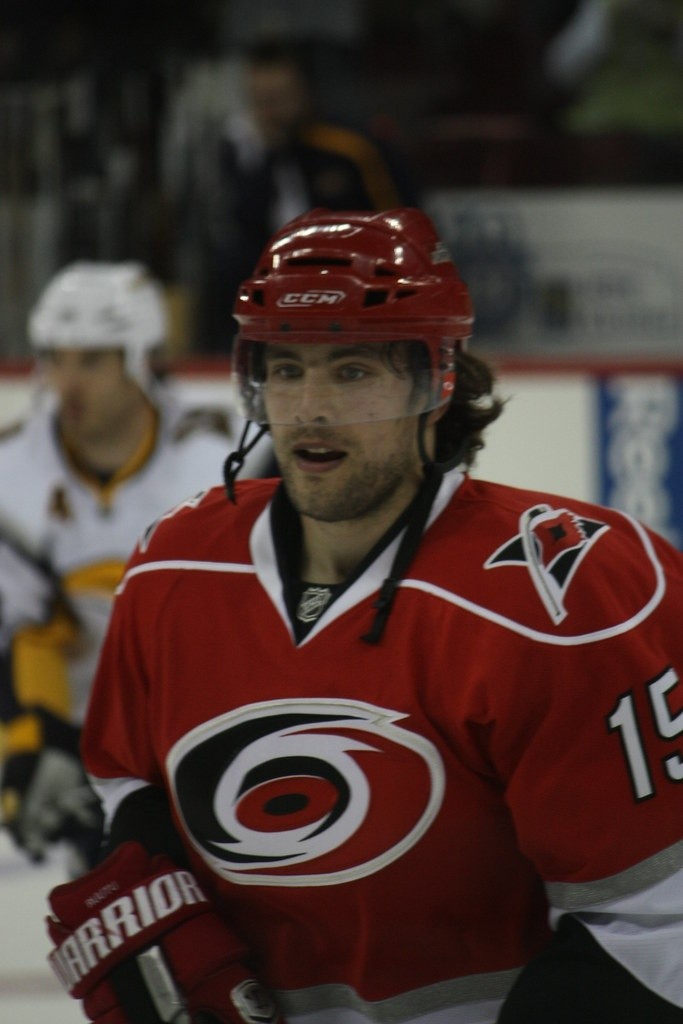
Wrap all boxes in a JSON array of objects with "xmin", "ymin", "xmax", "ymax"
[
  {"xmin": 231, "ymin": 204, "xmax": 473, "ymax": 428},
  {"xmin": 28, "ymin": 259, "xmax": 163, "ymax": 383}
]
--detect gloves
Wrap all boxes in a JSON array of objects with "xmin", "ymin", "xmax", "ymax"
[
  {"xmin": 45, "ymin": 839, "xmax": 287, "ymax": 1024},
  {"xmin": 15, "ymin": 748, "xmax": 107, "ymax": 866}
]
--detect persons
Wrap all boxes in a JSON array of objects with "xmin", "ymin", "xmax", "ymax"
[
  {"xmin": 195, "ymin": 65, "xmax": 373, "ymax": 364},
  {"xmin": 0, "ymin": 259, "xmax": 273, "ymax": 881},
  {"xmin": 560, "ymin": 0, "xmax": 683, "ymax": 186},
  {"xmin": 370, "ymin": 0, "xmax": 578, "ymax": 117},
  {"xmin": 50, "ymin": 204, "xmax": 683, "ymax": 1024}
]
